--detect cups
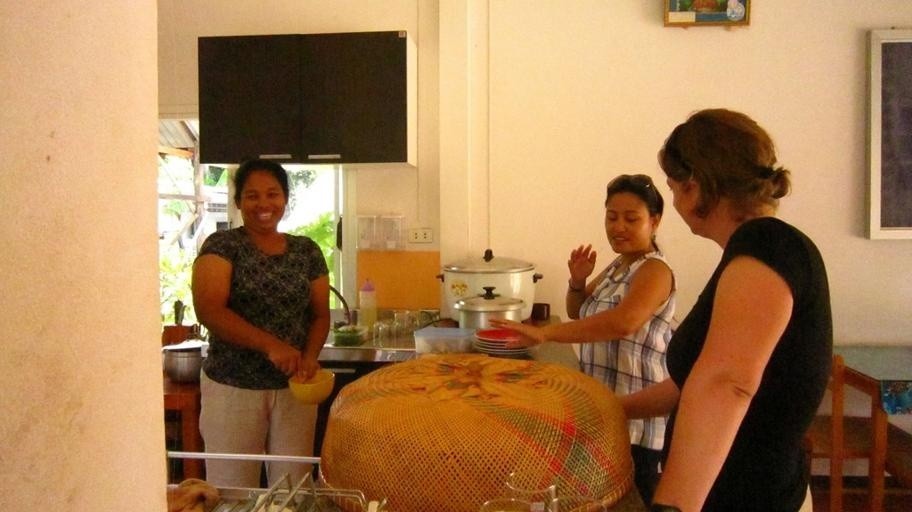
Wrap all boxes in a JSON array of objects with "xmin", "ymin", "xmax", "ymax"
[
  {"xmin": 482, "ymin": 471, "xmax": 606, "ymax": 512},
  {"xmin": 374, "ymin": 309, "xmax": 438, "ymax": 347},
  {"xmin": 534, "ymin": 304, "xmax": 549, "ymax": 319}
]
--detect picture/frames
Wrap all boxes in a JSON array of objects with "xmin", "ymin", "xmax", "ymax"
[{"xmin": 663, "ymin": 0, "xmax": 749, "ymax": 25}]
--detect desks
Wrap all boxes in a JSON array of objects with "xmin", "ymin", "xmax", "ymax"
[{"xmin": 829, "ymin": 344, "xmax": 911, "ymax": 508}]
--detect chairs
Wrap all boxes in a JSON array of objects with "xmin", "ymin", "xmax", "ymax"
[{"xmin": 804, "ymin": 353, "xmax": 875, "ymax": 509}]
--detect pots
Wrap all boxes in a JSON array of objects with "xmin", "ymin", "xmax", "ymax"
[{"xmin": 436, "ymin": 250, "xmax": 542, "ymax": 328}]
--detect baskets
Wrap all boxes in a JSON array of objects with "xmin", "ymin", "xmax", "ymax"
[{"xmin": 319, "ymin": 354, "xmax": 635, "ymax": 510}]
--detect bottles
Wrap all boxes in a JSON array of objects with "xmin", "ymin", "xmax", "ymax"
[{"xmin": 358, "ymin": 281, "xmax": 377, "ymax": 326}]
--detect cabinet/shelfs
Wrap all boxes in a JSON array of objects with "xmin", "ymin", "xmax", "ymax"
[{"xmin": 197, "ymin": 32, "xmax": 423, "ymax": 167}]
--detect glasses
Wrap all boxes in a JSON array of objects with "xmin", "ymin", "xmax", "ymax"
[{"xmin": 605, "ymin": 174, "xmax": 656, "ymax": 190}]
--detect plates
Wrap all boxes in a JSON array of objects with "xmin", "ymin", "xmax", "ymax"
[{"xmin": 472, "ymin": 329, "xmax": 530, "ymax": 355}]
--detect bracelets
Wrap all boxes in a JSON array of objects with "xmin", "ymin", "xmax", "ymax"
[{"xmin": 568, "ymin": 279, "xmax": 587, "ymax": 292}]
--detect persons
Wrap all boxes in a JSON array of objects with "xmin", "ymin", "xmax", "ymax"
[
  {"xmin": 191, "ymin": 157, "xmax": 331, "ymax": 488},
  {"xmin": 490, "ymin": 173, "xmax": 676, "ymax": 505},
  {"xmin": 617, "ymin": 106, "xmax": 834, "ymax": 512}
]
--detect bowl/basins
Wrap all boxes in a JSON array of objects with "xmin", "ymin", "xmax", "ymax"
[
  {"xmin": 289, "ymin": 371, "xmax": 337, "ymax": 404},
  {"xmin": 165, "ymin": 350, "xmax": 199, "ymax": 383}
]
